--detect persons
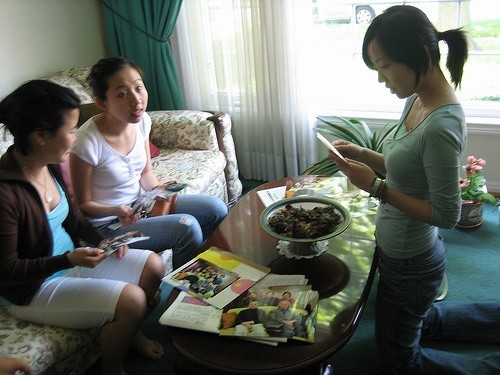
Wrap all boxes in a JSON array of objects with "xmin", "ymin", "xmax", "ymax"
[
  {"xmin": 261, "ymin": 291, "xmax": 280, "ymax": 306},
  {"xmin": 68, "ymin": 54, "xmax": 229, "ymax": 267},
  {"xmin": 238, "ymin": 292, "xmax": 260, "ymax": 306},
  {"xmin": 287, "ymin": 177, "xmax": 377, "ymax": 211},
  {"xmin": 234, "ymin": 301, "xmax": 266, "ymax": 337},
  {"xmin": 278, "ymin": 292, "xmax": 296, "ymax": 308},
  {"xmin": 181, "ymin": 265, "xmax": 226, "ymax": 296},
  {"xmin": 266, "ymin": 300, "xmax": 300, "ymax": 336},
  {"xmin": 0, "ymin": 79, "xmax": 167, "ymax": 375},
  {"xmin": 327, "ymin": 6, "xmax": 500, "ymax": 375}
]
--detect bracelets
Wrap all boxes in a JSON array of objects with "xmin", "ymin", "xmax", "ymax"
[{"xmin": 368, "ymin": 177, "xmax": 388, "ymax": 204}]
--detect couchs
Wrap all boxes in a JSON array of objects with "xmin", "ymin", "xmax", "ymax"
[{"xmin": 0, "ymin": 64, "xmax": 242, "ymax": 375}]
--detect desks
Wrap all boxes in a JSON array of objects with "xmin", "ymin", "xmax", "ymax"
[{"xmin": 165, "ymin": 175, "xmax": 379, "ymax": 375}]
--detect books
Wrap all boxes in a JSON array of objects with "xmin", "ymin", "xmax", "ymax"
[{"xmin": 159, "ymin": 290, "xmax": 287, "ymax": 346}]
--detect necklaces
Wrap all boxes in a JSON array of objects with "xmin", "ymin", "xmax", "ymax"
[{"xmin": 25, "ymin": 168, "xmax": 55, "ymax": 203}]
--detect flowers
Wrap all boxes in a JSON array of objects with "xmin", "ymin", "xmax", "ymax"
[{"xmin": 460, "ymin": 156, "xmax": 500, "ymax": 212}]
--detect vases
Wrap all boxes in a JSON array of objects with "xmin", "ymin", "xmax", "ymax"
[{"xmin": 456, "ymin": 200, "xmax": 483, "ymax": 229}]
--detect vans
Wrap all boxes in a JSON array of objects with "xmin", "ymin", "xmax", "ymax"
[{"xmin": 308, "ymin": 0, "xmax": 383, "ymax": 25}]
